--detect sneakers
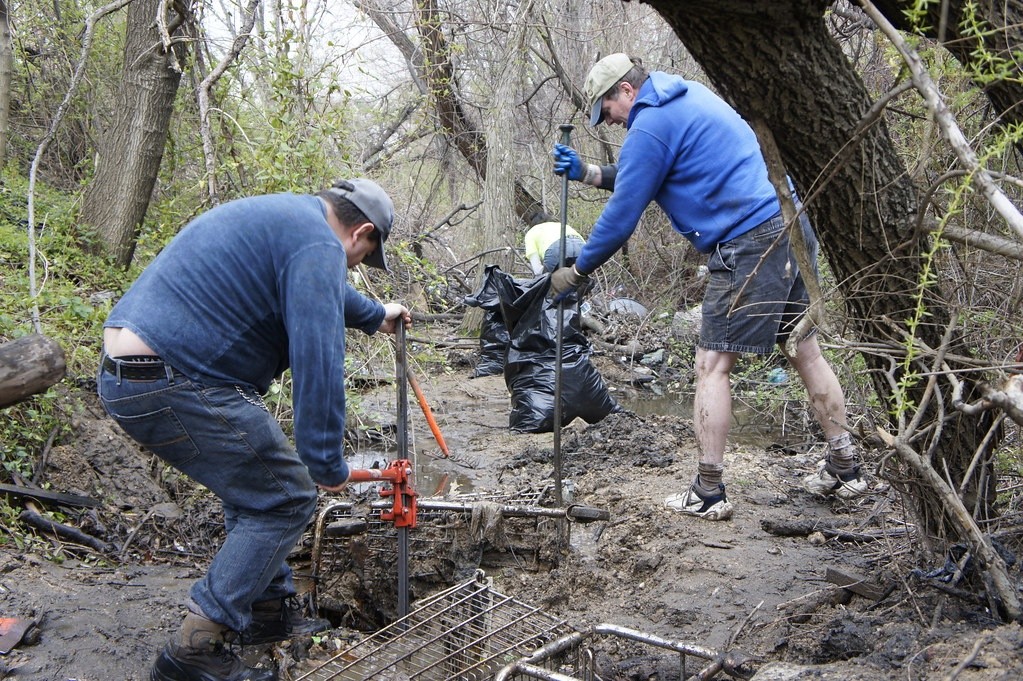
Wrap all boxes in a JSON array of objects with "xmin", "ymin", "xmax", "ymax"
[
  {"xmin": 802, "ymin": 455, "xmax": 867, "ymax": 499},
  {"xmin": 232, "ymin": 594, "xmax": 331, "ymax": 646},
  {"xmin": 150, "ymin": 610, "xmax": 278, "ymax": 681},
  {"xmin": 662, "ymin": 473, "xmax": 733, "ymax": 521}
]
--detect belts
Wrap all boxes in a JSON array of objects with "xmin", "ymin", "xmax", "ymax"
[{"xmin": 99, "ymin": 343, "xmax": 183, "ymax": 380}]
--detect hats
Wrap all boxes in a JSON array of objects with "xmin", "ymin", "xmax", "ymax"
[
  {"xmin": 584, "ymin": 52, "xmax": 636, "ymax": 129},
  {"xmin": 329, "ymin": 177, "xmax": 395, "ymax": 273}
]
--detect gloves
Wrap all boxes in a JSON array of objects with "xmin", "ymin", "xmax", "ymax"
[
  {"xmin": 552, "ymin": 143, "xmax": 592, "ymax": 185},
  {"xmin": 547, "ymin": 263, "xmax": 586, "ymax": 304}
]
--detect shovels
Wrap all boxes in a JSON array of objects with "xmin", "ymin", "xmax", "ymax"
[{"xmin": 536, "ymin": 122, "xmax": 577, "ymax": 552}]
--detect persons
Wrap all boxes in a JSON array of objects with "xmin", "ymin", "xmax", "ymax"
[
  {"xmin": 550, "ymin": 52, "xmax": 867, "ymax": 521},
  {"xmin": 525, "ymin": 210, "xmax": 586, "ymax": 276},
  {"xmin": 96, "ymin": 178, "xmax": 412, "ymax": 681}
]
